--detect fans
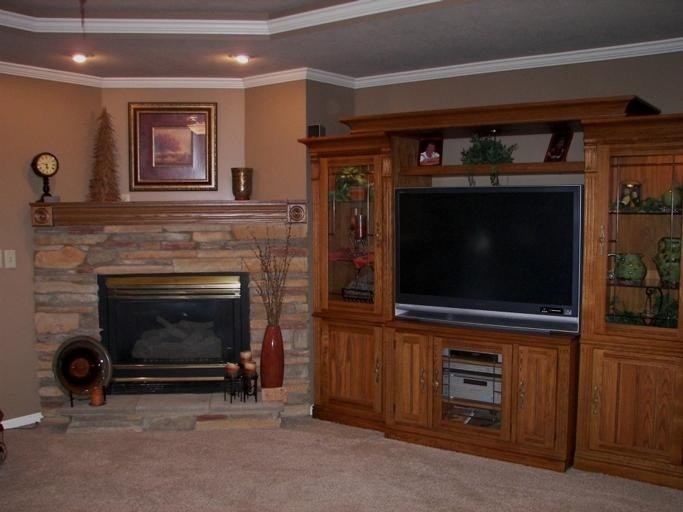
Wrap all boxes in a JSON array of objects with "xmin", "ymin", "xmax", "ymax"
[{"xmin": 52, "ymin": 334, "xmax": 113, "ymax": 408}]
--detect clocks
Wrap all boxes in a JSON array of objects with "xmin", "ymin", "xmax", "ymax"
[{"xmin": 30, "ymin": 153, "xmax": 58, "ymax": 204}]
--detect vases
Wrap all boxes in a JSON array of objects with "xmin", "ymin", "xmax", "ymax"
[
  {"xmin": 261, "ymin": 326, "xmax": 284, "ymax": 388},
  {"xmin": 232, "ymin": 169, "xmax": 252, "ymax": 199}
]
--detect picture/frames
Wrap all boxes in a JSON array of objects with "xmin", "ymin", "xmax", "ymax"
[{"xmin": 127, "ymin": 103, "xmax": 218, "ymax": 191}]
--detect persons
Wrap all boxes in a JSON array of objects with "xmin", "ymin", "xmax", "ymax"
[
  {"xmin": 419, "ymin": 142, "xmax": 441, "ymax": 166},
  {"xmin": 545, "ymin": 137, "xmax": 568, "ymax": 162}
]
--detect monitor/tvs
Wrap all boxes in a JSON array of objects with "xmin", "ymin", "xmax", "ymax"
[{"xmin": 394, "ymin": 183, "xmax": 583, "ymax": 335}]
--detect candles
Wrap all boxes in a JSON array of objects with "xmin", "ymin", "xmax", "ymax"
[{"xmin": 223, "ymin": 351, "xmax": 258, "ymax": 405}]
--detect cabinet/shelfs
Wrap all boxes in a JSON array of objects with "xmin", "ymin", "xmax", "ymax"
[
  {"xmin": 570, "ymin": 113, "xmax": 682, "ymax": 488},
  {"xmin": 382, "ymin": 317, "xmax": 578, "ymax": 476},
  {"xmin": 299, "ymin": 132, "xmax": 420, "ymax": 433}
]
